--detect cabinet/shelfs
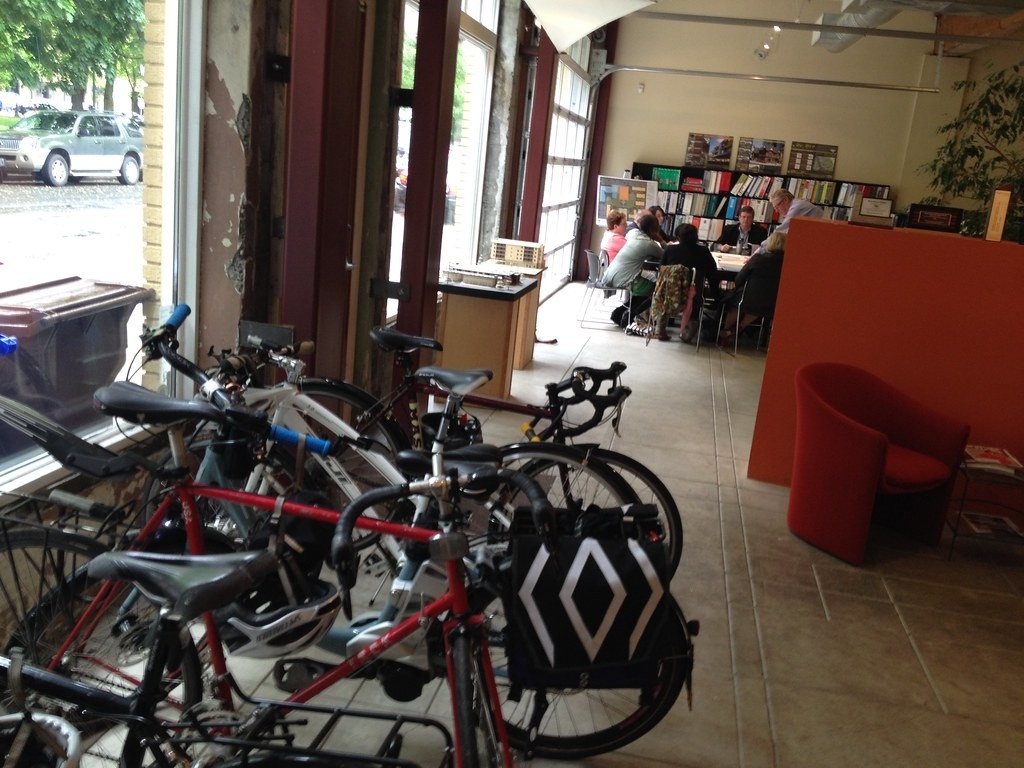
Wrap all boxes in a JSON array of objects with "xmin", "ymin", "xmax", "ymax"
[{"xmin": 631, "ymin": 161, "xmax": 891, "ymax": 243}]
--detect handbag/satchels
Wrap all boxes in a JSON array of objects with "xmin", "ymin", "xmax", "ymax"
[
  {"xmin": 506, "ymin": 506, "xmax": 691, "ymax": 692},
  {"xmin": 625, "ymin": 321, "xmax": 656, "ymax": 339}
]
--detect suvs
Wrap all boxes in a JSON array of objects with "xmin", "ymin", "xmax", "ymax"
[{"xmin": 0, "ymin": 110, "xmax": 144, "ymax": 188}]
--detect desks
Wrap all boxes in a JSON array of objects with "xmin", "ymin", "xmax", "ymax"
[
  {"xmin": 475, "ymin": 258, "xmax": 548, "ymax": 370},
  {"xmin": 711, "ymin": 252, "xmax": 752, "ymax": 280},
  {"xmin": 433, "ymin": 277, "xmax": 538, "ymax": 410}
]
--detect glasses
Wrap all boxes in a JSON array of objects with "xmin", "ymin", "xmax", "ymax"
[{"xmin": 773, "ymin": 199, "xmax": 785, "ymax": 210}]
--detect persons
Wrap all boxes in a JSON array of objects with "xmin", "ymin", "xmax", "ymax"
[
  {"xmin": 395, "ymin": 149, "xmax": 408, "ymax": 185},
  {"xmin": 0, "ymin": 94, "xmax": 146, "ymax": 117},
  {"xmin": 600, "ymin": 188, "xmax": 826, "ymax": 341}
]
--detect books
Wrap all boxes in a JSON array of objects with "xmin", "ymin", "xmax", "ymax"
[
  {"xmin": 645, "ymin": 168, "xmax": 889, "ymax": 290},
  {"xmin": 960, "ymin": 444, "xmax": 1024, "ymax": 538}
]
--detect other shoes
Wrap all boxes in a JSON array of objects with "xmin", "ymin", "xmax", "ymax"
[{"xmin": 719, "ymin": 325, "xmax": 742, "ymax": 345}]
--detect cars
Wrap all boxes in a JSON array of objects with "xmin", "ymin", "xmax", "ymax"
[{"xmin": 391, "ymin": 144, "xmax": 474, "ymax": 224}]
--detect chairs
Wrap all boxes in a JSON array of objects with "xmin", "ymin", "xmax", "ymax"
[{"xmin": 577, "ymin": 240, "xmax": 773, "ymax": 358}]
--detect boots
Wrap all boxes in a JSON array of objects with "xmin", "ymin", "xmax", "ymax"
[
  {"xmin": 611, "ymin": 296, "xmax": 653, "ymax": 329},
  {"xmin": 679, "ymin": 319, "xmax": 700, "ymax": 343},
  {"xmin": 654, "ymin": 316, "xmax": 672, "ymax": 341}
]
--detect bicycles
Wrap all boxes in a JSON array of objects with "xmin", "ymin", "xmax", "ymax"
[{"xmin": 2, "ymin": 302, "xmax": 702, "ymax": 768}]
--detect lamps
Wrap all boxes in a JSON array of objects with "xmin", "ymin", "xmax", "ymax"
[{"xmin": 755, "ymin": 41, "xmax": 774, "ymax": 60}]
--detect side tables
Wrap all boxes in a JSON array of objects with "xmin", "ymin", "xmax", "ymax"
[{"xmin": 946, "ymin": 458, "xmax": 1024, "ymax": 559}]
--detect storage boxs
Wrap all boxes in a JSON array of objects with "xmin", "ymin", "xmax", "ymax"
[{"xmin": 913, "ymin": 211, "xmax": 959, "ymax": 228}]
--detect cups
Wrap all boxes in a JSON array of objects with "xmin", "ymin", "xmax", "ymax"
[{"xmin": 511, "ymin": 273, "xmax": 520, "ymax": 285}]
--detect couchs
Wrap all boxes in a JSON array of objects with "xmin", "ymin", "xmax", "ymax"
[{"xmin": 786, "ymin": 361, "xmax": 971, "ymax": 567}]
